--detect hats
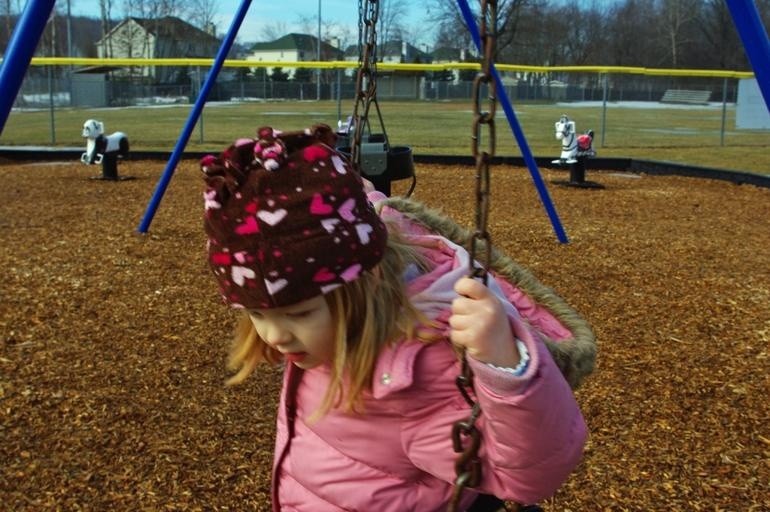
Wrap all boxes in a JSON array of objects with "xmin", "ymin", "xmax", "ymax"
[{"xmin": 201, "ymin": 123, "xmax": 386, "ymax": 308}]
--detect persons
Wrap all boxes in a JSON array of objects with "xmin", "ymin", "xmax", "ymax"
[{"xmin": 198, "ymin": 123, "xmax": 600, "ymax": 512}]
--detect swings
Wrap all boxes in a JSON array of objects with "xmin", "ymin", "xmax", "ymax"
[{"xmin": 347, "ymin": 0, "xmax": 499, "ymax": 512}]
